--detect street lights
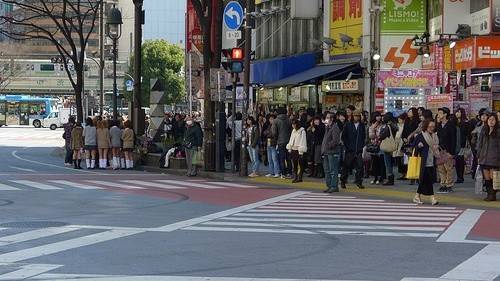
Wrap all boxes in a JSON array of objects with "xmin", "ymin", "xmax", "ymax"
[{"xmin": 103, "ymin": 3, "xmax": 123, "ymax": 123}]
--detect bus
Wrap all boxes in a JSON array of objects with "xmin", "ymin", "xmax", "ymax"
[
  {"xmin": 0, "ymin": 95, "xmax": 59, "ymax": 129},
  {"xmin": 108, "ymin": 106, "xmax": 151, "ymax": 120}
]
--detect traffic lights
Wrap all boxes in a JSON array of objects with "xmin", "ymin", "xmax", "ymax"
[
  {"xmin": 50, "ymin": 57, "xmax": 66, "ymax": 64},
  {"xmin": 231, "ymin": 61, "xmax": 243, "ymax": 73},
  {"xmin": 221, "ymin": 48, "xmax": 232, "ymax": 74},
  {"xmin": 231, "ymin": 48, "xmax": 243, "ymax": 60}
]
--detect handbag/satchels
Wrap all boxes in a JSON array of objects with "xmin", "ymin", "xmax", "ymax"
[
  {"xmin": 192, "ymin": 150, "xmax": 204, "ymax": 166},
  {"xmin": 406, "ymin": 147, "xmax": 421, "ymax": 179},
  {"xmin": 474, "ymin": 165, "xmax": 483, "ymax": 195},
  {"xmin": 366, "ymin": 143, "xmax": 378, "ymax": 153},
  {"xmin": 380, "ymin": 125, "xmax": 397, "ymax": 153},
  {"xmin": 362, "ymin": 145, "xmax": 372, "ymax": 161},
  {"xmin": 492, "ymin": 167, "xmax": 500, "ymax": 191},
  {"xmin": 436, "ymin": 148, "xmax": 448, "ymax": 166}
]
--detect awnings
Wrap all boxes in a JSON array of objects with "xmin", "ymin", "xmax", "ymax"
[{"xmin": 259, "ymin": 62, "xmax": 361, "ymax": 90}]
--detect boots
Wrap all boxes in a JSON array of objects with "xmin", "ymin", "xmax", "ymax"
[
  {"xmin": 383, "ymin": 176, "xmax": 394, "ymax": 185},
  {"xmin": 64, "ymin": 156, "xmax": 134, "ymax": 170}
]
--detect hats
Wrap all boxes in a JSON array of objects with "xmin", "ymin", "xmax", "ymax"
[
  {"xmin": 438, "ymin": 106, "xmax": 450, "ymax": 116},
  {"xmin": 353, "ymin": 110, "xmax": 362, "ymax": 116},
  {"xmin": 480, "ymin": 110, "xmax": 490, "ymax": 116}
]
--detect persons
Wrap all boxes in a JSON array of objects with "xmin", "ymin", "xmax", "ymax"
[{"xmin": 62, "ymin": 104, "xmax": 500, "ymax": 206}]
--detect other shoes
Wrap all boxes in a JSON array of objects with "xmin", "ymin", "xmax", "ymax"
[
  {"xmin": 443, "ymin": 188, "xmax": 452, "ymax": 194},
  {"xmin": 455, "ymin": 179, "xmax": 464, "ymax": 183},
  {"xmin": 413, "ymin": 197, "xmax": 423, "ymax": 204},
  {"xmin": 186, "ymin": 172, "xmax": 196, "ymax": 177},
  {"xmin": 432, "ymin": 198, "xmax": 438, "ymax": 206},
  {"xmin": 248, "ymin": 164, "xmax": 370, "ymax": 193},
  {"xmin": 371, "ymin": 179, "xmax": 378, "ymax": 184},
  {"xmin": 396, "ymin": 175, "xmax": 404, "ymax": 179},
  {"xmin": 376, "ymin": 178, "xmax": 383, "ymax": 184},
  {"xmin": 437, "ymin": 187, "xmax": 446, "ymax": 193}
]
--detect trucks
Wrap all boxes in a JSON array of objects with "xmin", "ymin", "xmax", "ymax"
[{"xmin": 42, "ymin": 107, "xmax": 73, "ymax": 130}]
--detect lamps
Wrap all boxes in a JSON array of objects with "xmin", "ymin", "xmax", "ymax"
[
  {"xmin": 437, "ymin": 34, "xmax": 479, "ymax": 49},
  {"xmin": 310, "ymin": 33, "xmax": 358, "ymax": 52},
  {"xmin": 411, "ymin": 30, "xmax": 434, "ymax": 57},
  {"xmin": 364, "ymin": 52, "xmax": 381, "ymax": 79}
]
